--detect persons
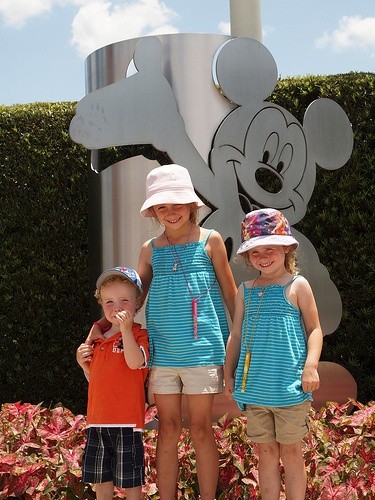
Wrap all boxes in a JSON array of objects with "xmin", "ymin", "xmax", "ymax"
[
  {"xmin": 86, "ymin": 164, "xmax": 238, "ymax": 500},
  {"xmin": 77, "ymin": 267, "xmax": 149, "ymax": 500},
  {"xmin": 223, "ymin": 208, "xmax": 322, "ymax": 500}
]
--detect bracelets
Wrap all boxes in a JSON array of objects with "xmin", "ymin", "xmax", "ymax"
[{"xmin": 92, "ymin": 322, "xmax": 111, "ymax": 331}]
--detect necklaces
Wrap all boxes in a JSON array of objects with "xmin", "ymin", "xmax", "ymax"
[
  {"xmin": 164, "ymin": 225, "xmax": 192, "ymax": 271},
  {"xmin": 256, "ymin": 278, "xmax": 276, "ymax": 296},
  {"xmin": 164, "ymin": 231, "xmax": 217, "ymax": 338}
]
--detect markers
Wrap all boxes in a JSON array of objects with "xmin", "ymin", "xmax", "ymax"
[
  {"xmin": 192, "ymin": 300, "xmax": 198, "ymax": 340},
  {"xmin": 241, "ymin": 352, "xmax": 251, "ymax": 393}
]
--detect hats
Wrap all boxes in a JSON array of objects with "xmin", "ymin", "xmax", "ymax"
[
  {"xmin": 136, "ymin": 161, "xmax": 205, "ymax": 218},
  {"xmin": 94, "ymin": 265, "xmax": 143, "ymax": 289},
  {"xmin": 236, "ymin": 207, "xmax": 299, "ymax": 256}
]
